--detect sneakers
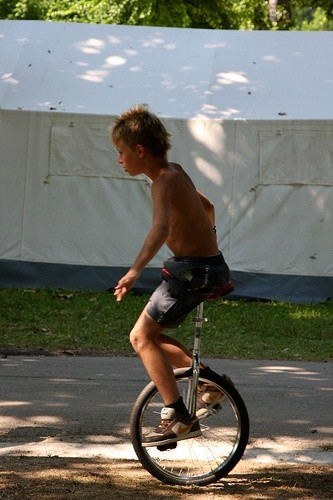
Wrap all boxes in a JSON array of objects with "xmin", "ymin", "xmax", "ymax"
[
  {"xmin": 194, "ymin": 374, "xmax": 239, "ymax": 416},
  {"xmin": 141, "ymin": 407, "xmax": 202, "ymax": 447}
]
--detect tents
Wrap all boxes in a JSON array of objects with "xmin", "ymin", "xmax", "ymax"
[{"xmin": 1, "ymin": 20, "xmax": 333, "ymax": 302}]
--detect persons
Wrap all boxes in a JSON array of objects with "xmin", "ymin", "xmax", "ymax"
[{"xmin": 110, "ymin": 107, "xmax": 230, "ymax": 447}]
